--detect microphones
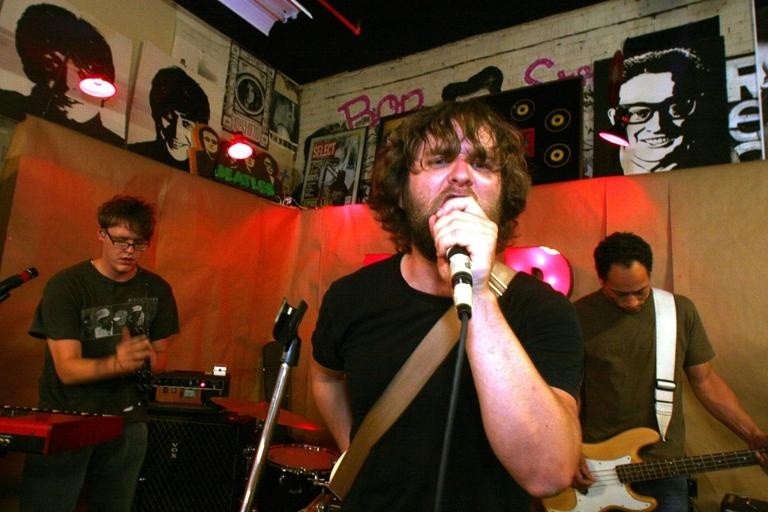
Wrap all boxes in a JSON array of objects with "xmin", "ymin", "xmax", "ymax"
[
  {"xmin": 444, "ymin": 244, "xmax": 472, "ymax": 320},
  {"xmin": 0, "ymin": 267, "xmax": 39, "ymax": 297}
]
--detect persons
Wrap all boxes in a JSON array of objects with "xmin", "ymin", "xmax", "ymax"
[
  {"xmin": 243, "ymin": 80, "xmax": 261, "ymax": 110},
  {"xmin": 309, "ymin": 95, "xmax": 586, "ymax": 512},
  {"xmin": 593, "ymin": 47, "xmax": 721, "ymax": 178},
  {"xmin": 0, "ymin": 3, "xmax": 126, "ymax": 149},
  {"xmin": 126, "ymin": 65, "xmax": 210, "ymax": 172},
  {"xmin": 215, "ymin": 138, "xmax": 284, "ymax": 203},
  {"xmin": 196, "ymin": 127, "xmax": 220, "ymax": 179},
  {"xmin": 566, "ymin": 229, "xmax": 768, "ymax": 512},
  {"xmin": 13, "ymin": 194, "xmax": 180, "ymax": 511},
  {"xmin": 292, "ymin": 120, "xmax": 348, "ymax": 208}
]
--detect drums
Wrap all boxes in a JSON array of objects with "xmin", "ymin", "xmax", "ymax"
[{"xmin": 247, "ymin": 444, "xmax": 342, "ymax": 512}]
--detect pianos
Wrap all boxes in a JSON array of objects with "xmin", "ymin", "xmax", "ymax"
[{"xmin": 0, "ymin": 405, "xmax": 123, "ymax": 455}]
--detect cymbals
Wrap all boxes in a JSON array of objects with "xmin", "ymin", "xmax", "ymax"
[{"xmin": 211, "ymin": 397, "xmax": 322, "ymax": 430}]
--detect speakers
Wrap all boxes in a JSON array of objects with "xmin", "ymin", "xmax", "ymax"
[{"xmin": 131, "ymin": 405, "xmax": 258, "ymax": 512}]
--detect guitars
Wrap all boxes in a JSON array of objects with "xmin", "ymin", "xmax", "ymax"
[{"xmin": 541, "ymin": 426, "xmax": 767, "ymax": 512}]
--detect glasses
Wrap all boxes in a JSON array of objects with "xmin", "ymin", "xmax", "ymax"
[
  {"xmin": 104, "ymin": 229, "xmax": 151, "ymax": 252},
  {"xmin": 616, "ymin": 93, "xmax": 706, "ymax": 125}
]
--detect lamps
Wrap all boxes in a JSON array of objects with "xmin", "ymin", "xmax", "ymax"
[
  {"xmin": 228, "ymin": 131, "xmax": 253, "ymax": 160},
  {"xmin": 218, "ymin": 0, "xmax": 314, "ymax": 36},
  {"xmin": 79, "ymin": 74, "xmax": 116, "ymax": 97},
  {"xmin": 599, "ymin": 108, "xmax": 630, "ymax": 145}
]
